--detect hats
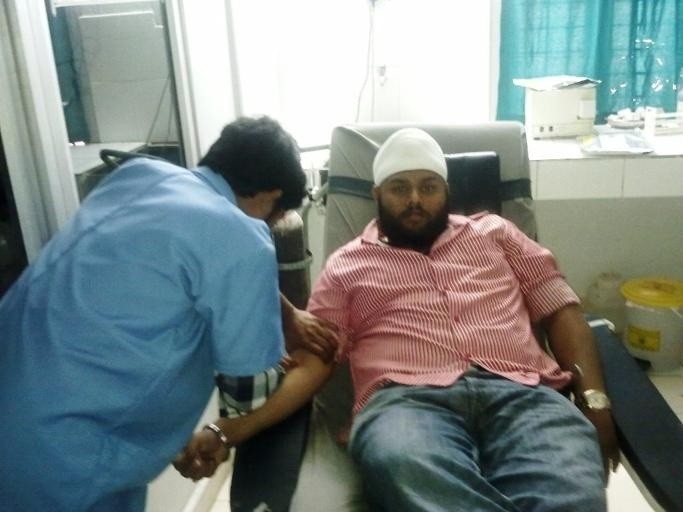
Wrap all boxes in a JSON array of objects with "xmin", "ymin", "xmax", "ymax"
[{"xmin": 372, "ymin": 127, "xmax": 449, "ymax": 187}]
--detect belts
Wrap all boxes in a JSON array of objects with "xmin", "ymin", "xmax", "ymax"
[{"xmin": 458, "ymin": 364, "xmax": 505, "ymax": 379}]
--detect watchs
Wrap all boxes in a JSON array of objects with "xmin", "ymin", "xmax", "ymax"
[{"xmin": 575, "ymin": 386, "xmax": 614, "ymax": 414}]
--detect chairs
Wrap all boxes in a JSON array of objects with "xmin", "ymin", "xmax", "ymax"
[{"xmin": 231, "ymin": 120, "xmax": 682, "ymax": 512}]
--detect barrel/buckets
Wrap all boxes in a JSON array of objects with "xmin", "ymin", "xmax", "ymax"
[{"xmin": 618, "ymin": 279, "xmax": 683, "ymax": 372}]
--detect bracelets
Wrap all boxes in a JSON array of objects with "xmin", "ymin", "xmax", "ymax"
[{"xmin": 203, "ymin": 422, "xmax": 232, "ymax": 463}]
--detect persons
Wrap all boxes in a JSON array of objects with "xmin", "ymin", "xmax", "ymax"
[
  {"xmin": 1, "ymin": 112, "xmax": 342, "ymax": 511},
  {"xmin": 171, "ymin": 127, "xmax": 625, "ymax": 512}
]
